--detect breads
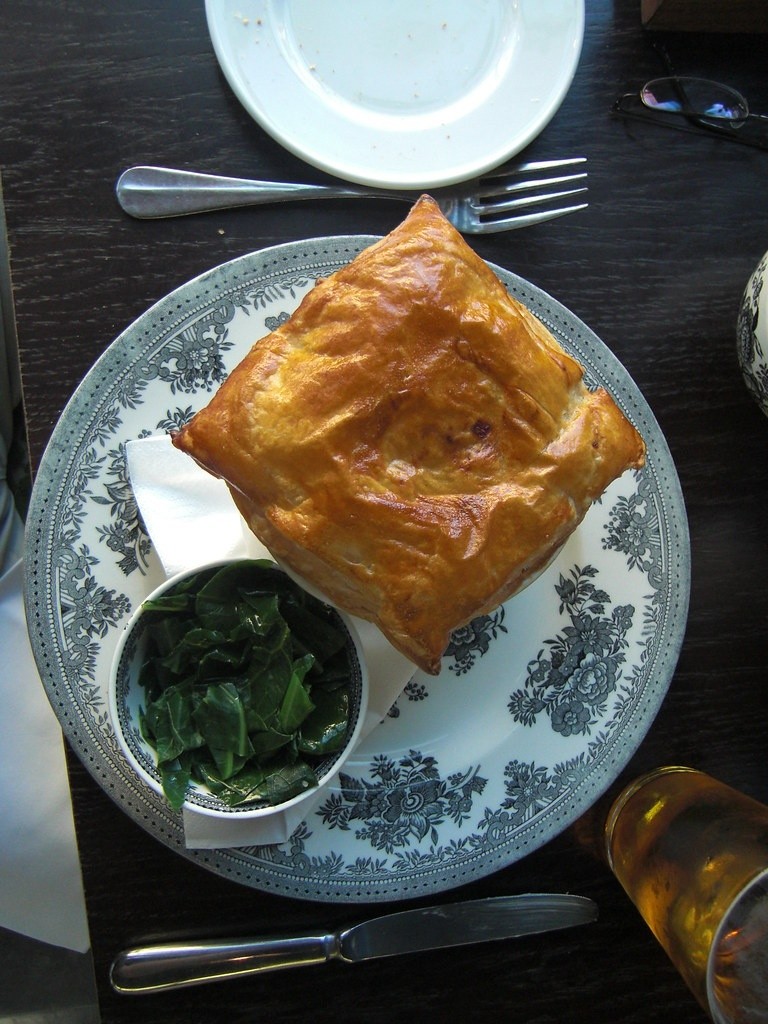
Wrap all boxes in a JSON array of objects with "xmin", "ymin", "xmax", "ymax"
[{"xmin": 166, "ymin": 193, "xmax": 645, "ymax": 676}]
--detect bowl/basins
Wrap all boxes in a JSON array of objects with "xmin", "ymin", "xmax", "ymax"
[
  {"xmin": 105, "ymin": 557, "xmax": 372, "ymax": 822},
  {"xmin": 735, "ymin": 249, "xmax": 768, "ymax": 415}
]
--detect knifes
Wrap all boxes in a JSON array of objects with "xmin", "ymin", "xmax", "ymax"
[{"xmin": 108, "ymin": 891, "xmax": 599, "ymax": 994}]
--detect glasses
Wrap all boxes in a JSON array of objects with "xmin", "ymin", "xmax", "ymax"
[{"xmin": 614, "ymin": 77, "xmax": 768, "ymax": 150}]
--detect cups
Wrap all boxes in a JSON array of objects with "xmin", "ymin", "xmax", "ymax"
[{"xmin": 601, "ymin": 764, "xmax": 768, "ymax": 1024}]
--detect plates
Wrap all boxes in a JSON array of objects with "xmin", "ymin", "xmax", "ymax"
[
  {"xmin": 22, "ymin": 235, "xmax": 692, "ymax": 903},
  {"xmin": 200, "ymin": 0, "xmax": 586, "ymax": 191}
]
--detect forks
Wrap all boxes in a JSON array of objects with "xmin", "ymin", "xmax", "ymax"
[{"xmin": 115, "ymin": 157, "xmax": 589, "ymax": 235}]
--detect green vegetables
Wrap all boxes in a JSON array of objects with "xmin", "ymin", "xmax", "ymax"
[{"xmin": 138, "ymin": 559, "xmax": 352, "ymax": 811}]
